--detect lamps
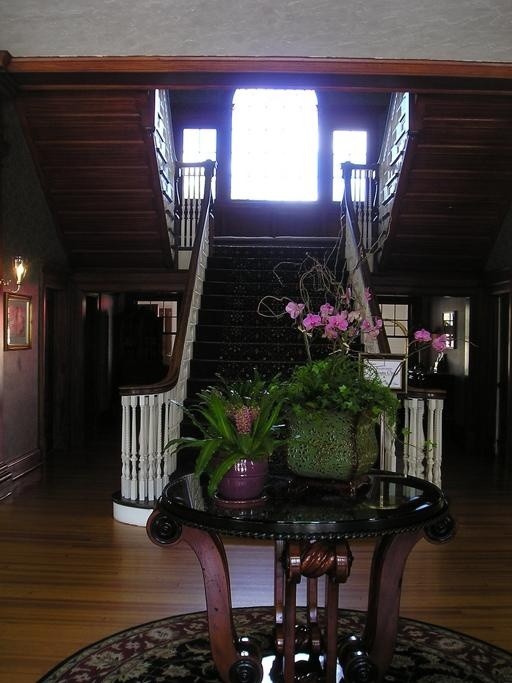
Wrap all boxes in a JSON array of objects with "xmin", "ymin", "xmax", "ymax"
[{"xmin": 0, "ymin": 252, "xmax": 29, "ymax": 293}]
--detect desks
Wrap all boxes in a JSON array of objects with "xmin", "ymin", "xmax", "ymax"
[{"xmin": 146, "ymin": 471, "xmax": 457, "ymax": 683}]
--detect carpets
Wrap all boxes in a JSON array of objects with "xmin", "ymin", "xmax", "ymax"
[{"xmin": 35, "ymin": 606, "xmax": 512, "ymax": 683}]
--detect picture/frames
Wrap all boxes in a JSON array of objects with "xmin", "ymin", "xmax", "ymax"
[
  {"xmin": 4, "ymin": 292, "xmax": 32, "ymax": 351},
  {"xmin": 442, "ymin": 310, "xmax": 456, "ymax": 350}
]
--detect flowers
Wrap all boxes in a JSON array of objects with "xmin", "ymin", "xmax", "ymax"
[
  {"xmin": 255, "ymin": 206, "xmax": 477, "ymax": 410},
  {"xmin": 152, "ymin": 367, "xmax": 298, "ymax": 499}
]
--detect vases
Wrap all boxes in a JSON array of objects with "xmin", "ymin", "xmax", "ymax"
[
  {"xmin": 287, "ymin": 405, "xmax": 379, "ymax": 497},
  {"xmin": 211, "ymin": 457, "xmax": 267, "ymax": 509}
]
